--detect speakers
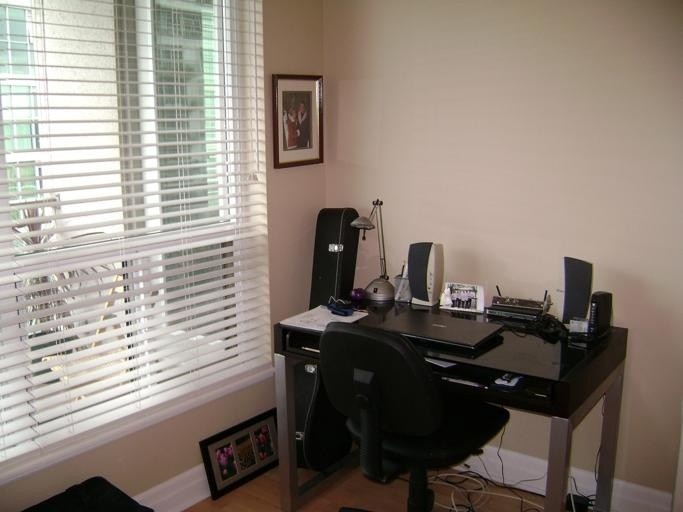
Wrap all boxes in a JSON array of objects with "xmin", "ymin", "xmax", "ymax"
[
  {"xmin": 409, "ymin": 242, "xmax": 444, "ymax": 306},
  {"xmin": 562, "ymin": 256, "xmax": 592, "ymax": 326}
]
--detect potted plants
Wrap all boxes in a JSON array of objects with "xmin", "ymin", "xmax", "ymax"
[{"xmin": 12, "ymin": 199, "xmax": 112, "ymax": 384}]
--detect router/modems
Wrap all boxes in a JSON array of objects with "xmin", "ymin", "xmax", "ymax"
[{"xmin": 492, "ymin": 285, "xmax": 548, "ymax": 310}]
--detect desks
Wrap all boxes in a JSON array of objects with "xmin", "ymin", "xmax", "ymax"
[
  {"xmin": 272, "ymin": 291, "xmax": 627, "ymax": 511},
  {"xmin": 20, "ymin": 475, "xmax": 155, "ymax": 511}
]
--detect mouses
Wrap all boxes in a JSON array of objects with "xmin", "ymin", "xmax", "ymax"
[{"xmin": 495, "ymin": 373, "xmax": 524, "ymax": 386}]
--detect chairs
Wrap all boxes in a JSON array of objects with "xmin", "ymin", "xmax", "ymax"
[{"xmin": 299, "ymin": 318, "xmax": 512, "ymax": 511}]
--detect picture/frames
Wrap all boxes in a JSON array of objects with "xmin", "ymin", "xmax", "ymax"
[
  {"xmin": 271, "ymin": 72, "xmax": 323, "ymax": 169},
  {"xmin": 197, "ymin": 407, "xmax": 281, "ymax": 501}
]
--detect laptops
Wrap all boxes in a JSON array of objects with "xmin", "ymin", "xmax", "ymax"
[{"xmin": 375, "ymin": 310, "xmax": 504, "ymax": 353}]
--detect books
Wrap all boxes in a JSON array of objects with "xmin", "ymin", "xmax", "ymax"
[
  {"xmin": 440, "ymin": 363, "xmax": 492, "ymax": 390},
  {"xmin": 301, "ymin": 338, "xmax": 320, "ymax": 354}
]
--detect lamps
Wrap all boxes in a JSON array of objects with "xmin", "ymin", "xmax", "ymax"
[{"xmin": 349, "ymin": 197, "xmax": 396, "ymax": 306}]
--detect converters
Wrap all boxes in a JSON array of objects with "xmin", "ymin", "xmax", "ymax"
[{"xmin": 567, "ymin": 493, "xmax": 588, "ymax": 512}]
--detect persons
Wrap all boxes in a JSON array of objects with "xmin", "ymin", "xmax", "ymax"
[
  {"xmin": 451, "ymin": 289, "xmax": 475, "ymax": 308},
  {"xmin": 281, "ymin": 101, "xmax": 312, "ymax": 151}
]
